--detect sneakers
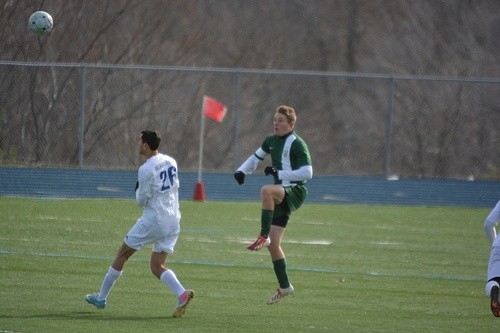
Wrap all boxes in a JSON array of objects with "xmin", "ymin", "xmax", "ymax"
[
  {"xmin": 248, "ymin": 236, "xmax": 271, "ymax": 250},
  {"xmin": 172, "ymin": 290, "xmax": 195, "ymax": 317},
  {"xmin": 266, "ymin": 283, "xmax": 294, "ymax": 304},
  {"xmin": 86, "ymin": 293, "xmax": 106, "ymax": 310}
]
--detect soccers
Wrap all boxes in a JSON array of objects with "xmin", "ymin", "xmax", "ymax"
[{"xmin": 28, "ymin": 11, "xmax": 54, "ymax": 36}]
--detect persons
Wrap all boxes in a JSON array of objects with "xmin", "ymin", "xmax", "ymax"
[
  {"xmin": 83, "ymin": 131, "xmax": 195, "ymax": 318},
  {"xmin": 483, "ymin": 198, "xmax": 500, "ymax": 317},
  {"xmin": 233, "ymin": 105, "xmax": 313, "ymax": 301}
]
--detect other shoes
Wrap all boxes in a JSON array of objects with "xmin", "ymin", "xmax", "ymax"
[{"xmin": 491, "ymin": 285, "xmax": 500, "ymax": 317}]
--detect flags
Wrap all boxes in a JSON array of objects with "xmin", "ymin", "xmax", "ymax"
[{"xmin": 204, "ymin": 98, "xmax": 225, "ymax": 122}]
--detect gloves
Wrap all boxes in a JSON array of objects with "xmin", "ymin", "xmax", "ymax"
[
  {"xmin": 234, "ymin": 171, "xmax": 245, "ymax": 184},
  {"xmin": 265, "ymin": 167, "xmax": 278, "ymax": 179}
]
